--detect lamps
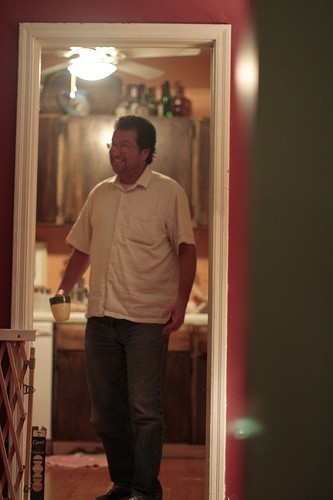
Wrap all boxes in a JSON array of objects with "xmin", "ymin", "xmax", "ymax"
[{"xmin": 64, "ymin": 46, "xmax": 120, "ymax": 99}]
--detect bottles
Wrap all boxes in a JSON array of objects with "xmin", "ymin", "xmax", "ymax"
[{"xmin": 111, "ymin": 79, "xmax": 184, "ymax": 117}]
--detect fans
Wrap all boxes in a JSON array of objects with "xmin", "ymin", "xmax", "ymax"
[{"xmin": 40, "ymin": 46, "xmax": 201, "ymax": 80}]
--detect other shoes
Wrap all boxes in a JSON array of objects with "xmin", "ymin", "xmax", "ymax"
[
  {"xmin": 126, "ymin": 489, "xmax": 148, "ymax": 500},
  {"xmin": 94, "ymin": 483, "xmax": 130, "ymax": 500}
]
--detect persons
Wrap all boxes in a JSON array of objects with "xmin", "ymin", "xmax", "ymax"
[{"xmin": 55, "ymin": 115, "xmax": 196, "ymax": 500}]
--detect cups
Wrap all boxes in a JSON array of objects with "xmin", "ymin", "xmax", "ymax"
[{"xmin": 49, "ymin": 296, "xmax": 71, "ymax": 322}]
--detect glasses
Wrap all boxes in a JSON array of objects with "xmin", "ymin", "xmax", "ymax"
[{"xmin": 107, "ymin": 143, "xmax": 130, "ymax": 151}]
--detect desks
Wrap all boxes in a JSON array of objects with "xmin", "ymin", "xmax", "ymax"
[{"xmin": 52, "ymin": 311, "xmax": 207, "ymax": 443}]
